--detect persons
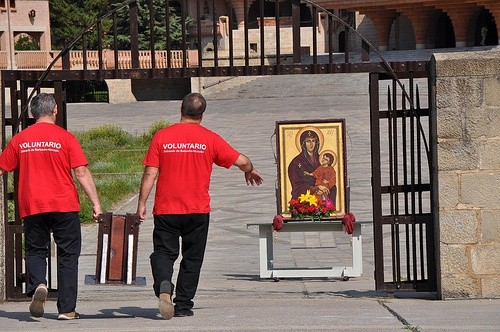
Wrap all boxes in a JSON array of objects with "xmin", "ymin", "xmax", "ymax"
[
  {"xmin": 0, "ymin": 94, "xmax": 103, "ymax": 320},
  {"xmin": 135, "ymin": 93, "xmax": 264, "ymax": 319}
]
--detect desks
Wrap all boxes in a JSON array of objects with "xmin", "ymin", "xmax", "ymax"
[{"xmin": 247, "ymin": 219, "xmax": 372, "ymax": 282}]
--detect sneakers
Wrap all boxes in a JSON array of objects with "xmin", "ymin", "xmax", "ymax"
[
  {"xmin": 29, "ymin": 284, "xmax": 47, "ymax": 317},
  {"xmin": 58, "ymin": 311, "xmax": 79, "ymax": 319}
]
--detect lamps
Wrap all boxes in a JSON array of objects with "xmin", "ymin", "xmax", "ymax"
[{"xmin": 27, "ymin": 8, "xmax": 38, "ymax": 23}]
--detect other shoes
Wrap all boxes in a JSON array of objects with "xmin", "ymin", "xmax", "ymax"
[
  {"xmin": 175, "ymin": 311, "xmax": 193, "ymax": 316},
  {"xmin": 158, "ymin": 280, "xmax": 174, "ymax": 320}
]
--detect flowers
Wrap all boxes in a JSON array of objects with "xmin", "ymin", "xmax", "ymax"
[{"xmin": 283, "ymin": 190, "xmax": 338, "ymax": 223}]
